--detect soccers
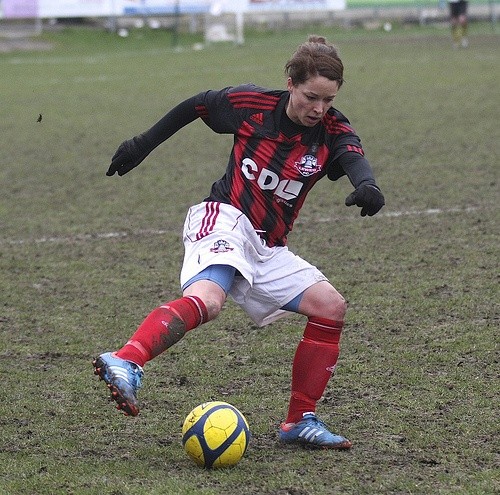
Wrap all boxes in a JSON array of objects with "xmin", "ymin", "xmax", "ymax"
[{"xmin": 182, "ymin": 401, "xmax": 250, "ymax": 468}]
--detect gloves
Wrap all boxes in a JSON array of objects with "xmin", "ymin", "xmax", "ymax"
[
  {"xmin": 344, "ymin": 183, "xmax": 385, "ymax": 217},
  {"xmin": 106, "ymin": 134, "xmax": 147, "ymax": 176}
]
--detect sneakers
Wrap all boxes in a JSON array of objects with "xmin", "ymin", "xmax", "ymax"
[
  {"xmin": 92, "ymin": 352, "xmax": 144, "ymax": 417},
  {"xmin": 278, "ymin": 412, "xmax": 352, "ymax": 448}
]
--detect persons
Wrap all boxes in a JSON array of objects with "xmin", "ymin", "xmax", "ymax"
[
  {"xmin": 92, "ymin": 35, "xmax": 386, "ymax": 449},
  {"xmin": 450, "ymin": 0, "xmax": 470, "ymax": 50}
]
